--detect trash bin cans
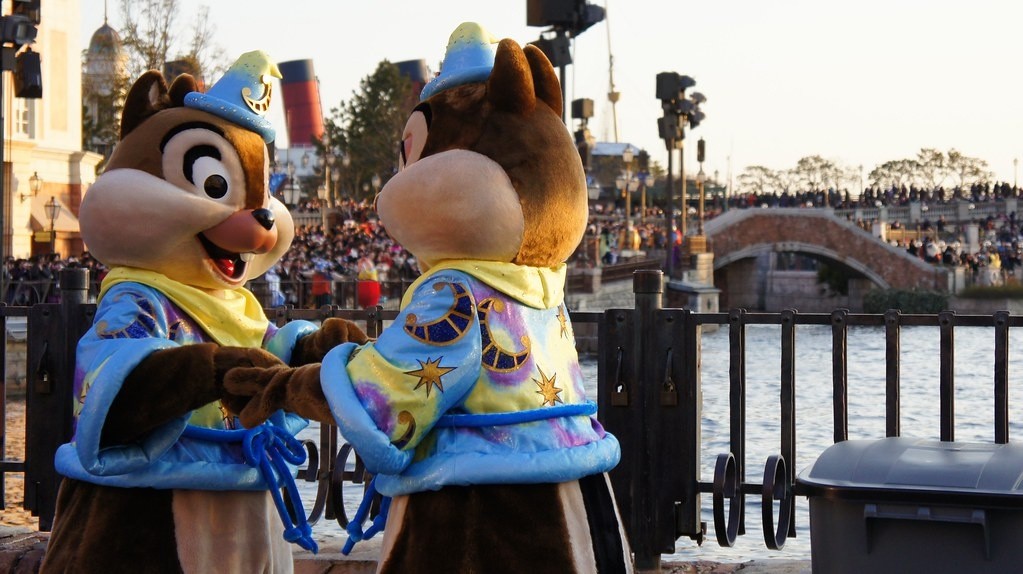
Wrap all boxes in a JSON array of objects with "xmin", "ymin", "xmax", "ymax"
[{"xmin": 794, "ymin": 437, "xmax": 1023, "ymax": 574}]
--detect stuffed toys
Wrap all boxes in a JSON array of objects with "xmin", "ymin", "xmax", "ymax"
[
  {"xmin": 38, "ymin": 51, "xmax": 368, "ymax": 574},
  {"xmin": 223, "ymin": 23, "xmax": 635, "ymax": 574}
]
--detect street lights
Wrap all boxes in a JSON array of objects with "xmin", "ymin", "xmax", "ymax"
[
  {"xmin": 623, "ymin": 143, "xmax": 634, "ymax": 252},
  {"xmin": 44, "ymin": 196, "xmax": 62, "ymax": 254}
]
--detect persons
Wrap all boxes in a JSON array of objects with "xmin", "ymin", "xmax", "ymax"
[
  {"xmin": 252, "ymin": 193, "xmax": 420, "ymax": 308},
  {"xmin": 2, "ymin": 252, "xmax": 108, "ymax": 304},
  {"xmin": 847, "ymin": 211, "xmax": 1023, "ymax": 285},
  {"xmin": 572, "ymin": 193, "xmax": 722, "ymax": 268},
  {"xmin": 729, "ymin": 181, "xmax": 1023, "ymax": 211}
]
